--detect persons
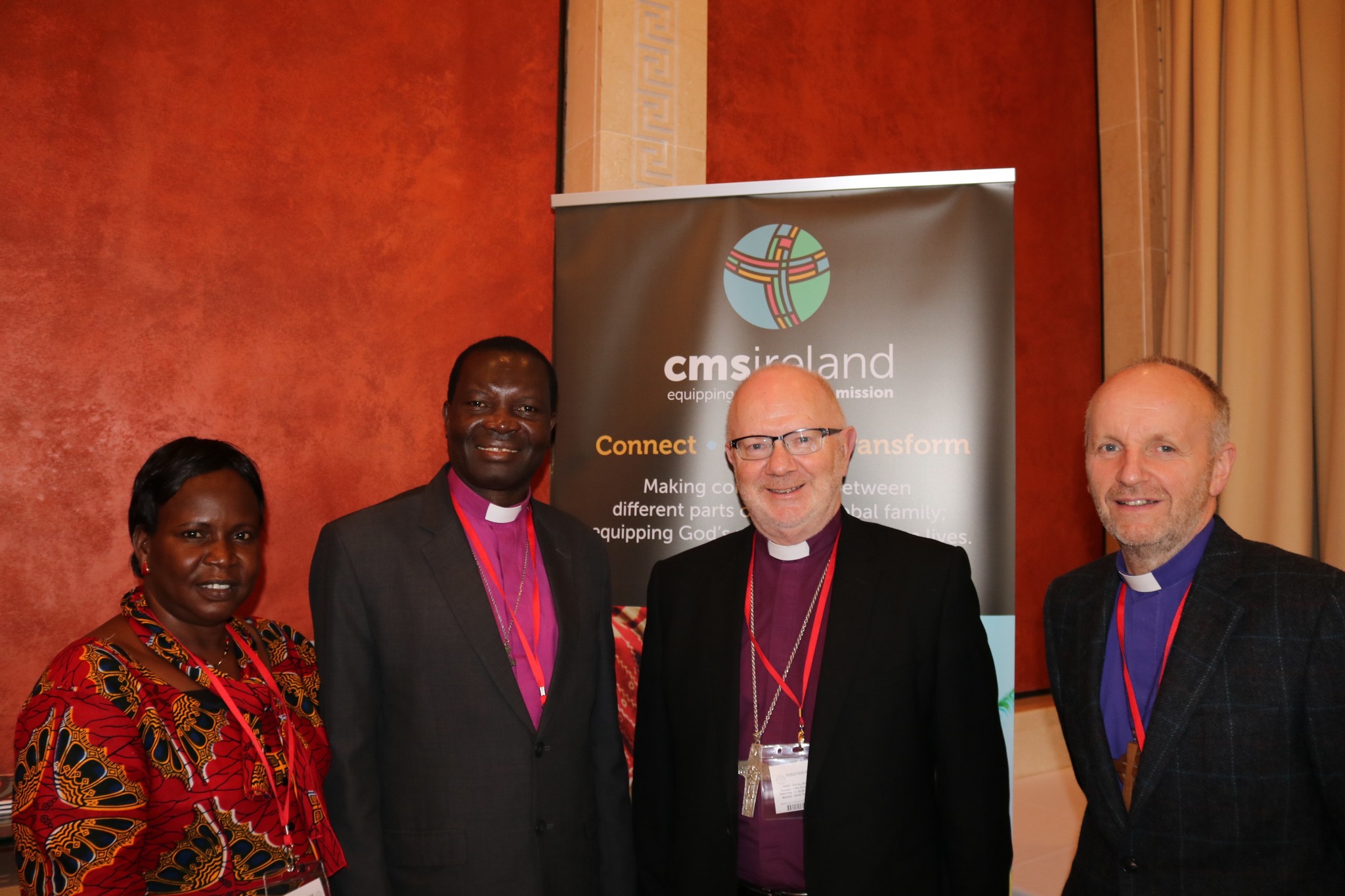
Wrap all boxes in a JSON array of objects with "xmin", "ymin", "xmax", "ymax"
[
  {"xmin": 1043, "ymin": 355, "xmax": 1345, "ymax": 896},
  {"xmin": 631, "ymin": 362, "xmax": 1014, "ymax": 896},
  {"xmin": 11, "ymin": 433, "xmax": 346, "ymax": 896},
  {"xmin": 306, "ymin": 332, "xmax": 638, "ymax": 896}
]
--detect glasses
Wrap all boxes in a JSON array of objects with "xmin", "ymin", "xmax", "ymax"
[{"xmin": 725, "ymin": 428, "xmax": 841, "ymax": 461}]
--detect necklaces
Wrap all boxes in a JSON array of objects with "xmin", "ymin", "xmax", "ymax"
[
  {"xmin": 1114, "ymin": 640, "xmax": 1164, "ymax": 814},
  {"xmin": 204, "ymin": 632, "xmax": 230, "ymax": 666},
  {"xmin": 738, "ymin": 547, "xmax": 833, "ymax": 821},
  {"xmin": 469, "ymin": 504, "xmax": 529, "ymax": 664}
]
